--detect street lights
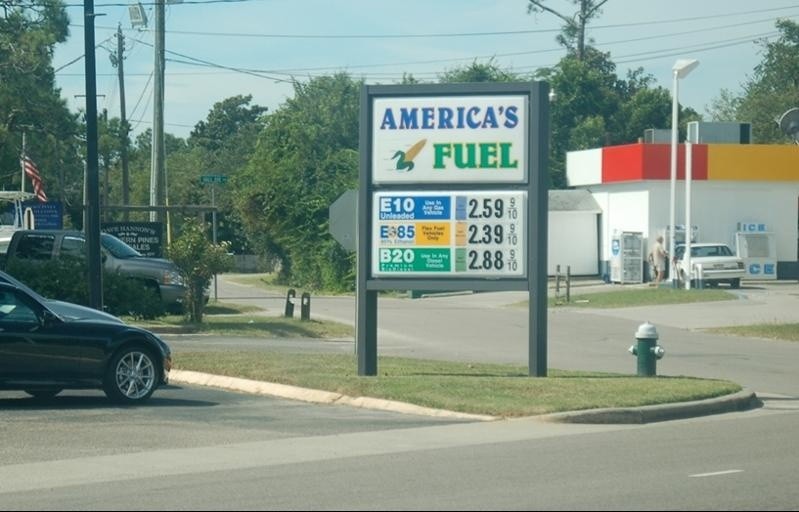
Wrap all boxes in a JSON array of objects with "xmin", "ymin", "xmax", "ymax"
[{"xmin": 668, "ymin": 58, "xmax": 699, "ymax": 289}]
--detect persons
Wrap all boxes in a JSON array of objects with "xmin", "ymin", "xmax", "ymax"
[{"xmin": 654, "ymin": 235, "xmax": 669, "ymax": 289}]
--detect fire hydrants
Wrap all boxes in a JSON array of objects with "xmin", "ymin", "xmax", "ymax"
[{"xmin": 628, "ymin": 321, "xmax": 665, "ymax": 378}]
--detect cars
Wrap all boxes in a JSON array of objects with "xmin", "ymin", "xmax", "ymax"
[
  {"xmin": 0, "ymin": 229, "xmax": 187, "ymax": 312},
  {"xmin": 674, "ymin": 243, "xmax": 745, "ymax": 290},
  {"xmin": 0, "ymin": 269, "xmax": 173, "ymax": 407}
]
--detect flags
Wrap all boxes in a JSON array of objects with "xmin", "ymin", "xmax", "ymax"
[{"xmin": 19, "ymin": 156, "xmax": 47, "ymax": 204}]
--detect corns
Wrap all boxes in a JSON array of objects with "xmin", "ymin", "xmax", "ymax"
[{"xmin": 392, "ymin": 139, "xmax": 427, "ymax": 171}]
